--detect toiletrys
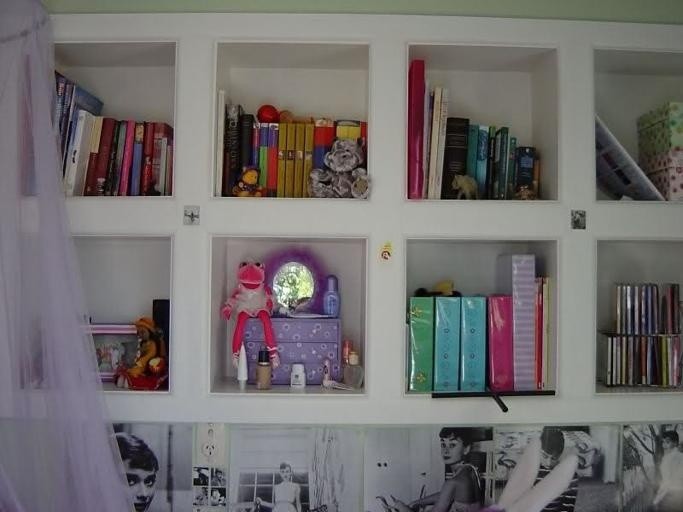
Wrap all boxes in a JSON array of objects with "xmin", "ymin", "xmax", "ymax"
[
  {"xmin": 255, "ymin": 350, "xmax": 272, "ymax": 390},
  {"xmin": 344, "ymin": 352, "xmax": 363, "ymax": 388},
  {"xmin": 323, "ymin": 275, "xmax": 340, "ymax": 316},
  {"xmin": 235, "ymin": 339, "xmax": 250, "ymax": 390}
]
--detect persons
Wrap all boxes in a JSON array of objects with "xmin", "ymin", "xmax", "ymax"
[
  {"xmin": 190, "ymin": 468, "xmax": 227, "ymax": 507},
  {"xmin": 532, "ymin": 429, "xmax": 577, "ymax": 511},
  {"xmin": 377, "ymin": 429, "xmax": 484, "ymax": 511},
  {"xmin": 254, "ymin": 463, "xmax": 301, "ymax": 512},
  {"xmin": 650, "ymin": 428, "xmax": 683, "ymax": 511},
  {"xmin": 102, "ymin": 432, "xmax": 158, "ymax": 512}
]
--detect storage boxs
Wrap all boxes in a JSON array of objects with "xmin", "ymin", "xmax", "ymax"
[
  {"xmin": 245, "ymin": 315, "xmax": 342, "ymax": 384},
  {"xmin": 636, "ymin": 98, "xmax": 683, "ymax": 156},
  {"xmin": 636, "ymin": 147, "xmax": 683, "ymax": 202},
  {"xmin": 75, "ymin": 323, "xmax": 137, "ymax": 382}
]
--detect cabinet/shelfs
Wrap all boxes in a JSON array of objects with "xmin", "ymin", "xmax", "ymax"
[{"xmin": 0, "ymin": 14, "xmax": 683, "ymax": 426}]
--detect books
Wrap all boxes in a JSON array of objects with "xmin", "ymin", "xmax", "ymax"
[
  {"xmin": 26, "ymin": 69, "xmax": 173, "ymax": 196},
  {"xmin": 215, "ymin": 89, "xmax": 254, "ymax": 196},
  {"xmin": 251, "ymin": 123, "xmax": 313, "ymax": 198},
  {"xmin": 409, "ymin": 58, "xmax": 540, "ymax": 201},
  {"xmin": 596, "ymin": 281, "xmax": 682, "ymax": 387},
  {"xmin": 314, "ymin": 119, "xmax": 367, "ymax": 167}
]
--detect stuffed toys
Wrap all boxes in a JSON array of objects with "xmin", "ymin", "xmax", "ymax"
[
  {"xmin": 232, "ymin": 165, "xmax": 262, "ymax": 198},
  {"xmin": 217, "ymin": 259, "xmax": 281, "ymax": 370},
  {"xmin": 309, "ymin": 137, "xmax": 369, "ymax": 198}
]
